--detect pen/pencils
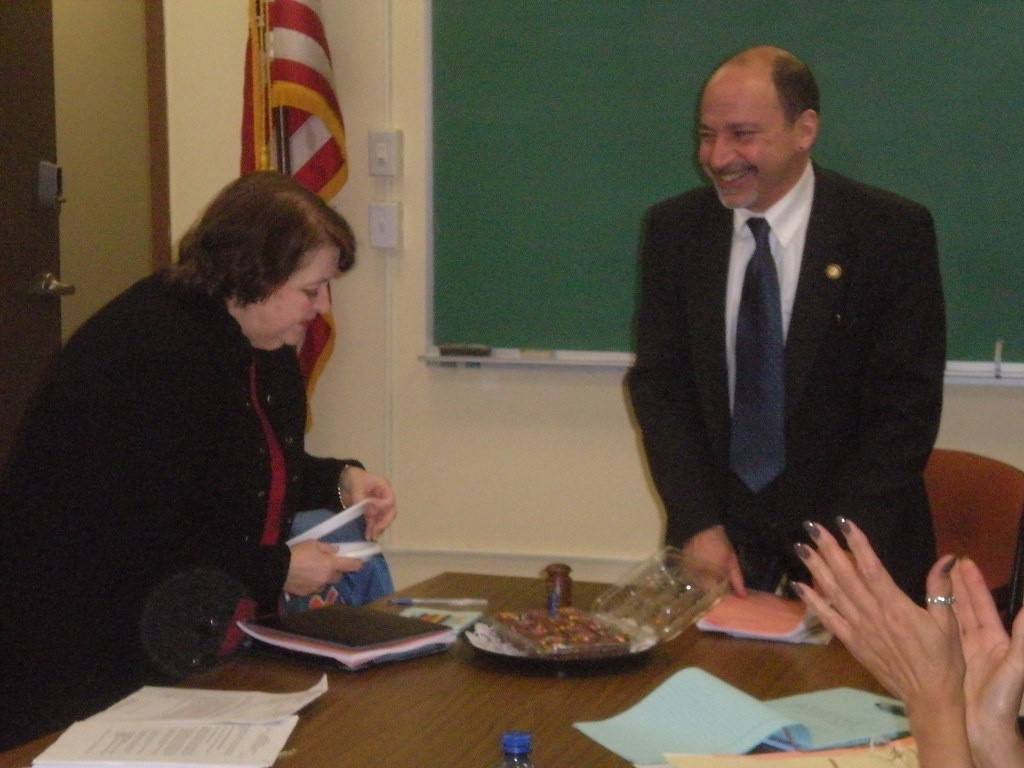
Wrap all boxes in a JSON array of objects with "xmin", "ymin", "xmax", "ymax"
[{"xmin": 389, "ymin": 598, "xmax": 489, "ymax": 607}]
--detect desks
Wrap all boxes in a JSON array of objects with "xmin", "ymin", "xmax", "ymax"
[{"xmin": 0, "ymin": 571, "xmax": 911, "ymax": 768}]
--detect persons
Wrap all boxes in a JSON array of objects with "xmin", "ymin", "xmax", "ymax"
[
  {"xmin": 790, "ymin": 515, "xmax": 1024, "ymax": 768},
  {"xmin": 623, "ymin": 46, "xmax": 947, "ymax": 618},
  {"xmin": 2, "ymin": 172, "xmax": 396, "ymax": 750}
]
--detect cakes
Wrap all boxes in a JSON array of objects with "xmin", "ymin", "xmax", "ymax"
[{"xmin": 494, "ymin": 607, "xmax": 632, "ymax": 657}]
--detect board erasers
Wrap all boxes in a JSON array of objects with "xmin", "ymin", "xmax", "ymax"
[{"xmin": 438, "ymin": 345, "xmax": 491, "ymax": 357}]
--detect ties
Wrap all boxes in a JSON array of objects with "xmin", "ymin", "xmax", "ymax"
[{"xmin": 728, "ymin": 218, "xmax": 785, "ymax": 493}]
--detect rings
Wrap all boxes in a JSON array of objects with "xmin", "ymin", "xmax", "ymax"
[{"xmin": 927, "ymin": 597, "xmax": 958, "ymax": 605}]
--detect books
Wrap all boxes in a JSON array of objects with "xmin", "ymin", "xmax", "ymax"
[
  {"xmin": 237, "ymin": 603, "xmax": 455, "ymax": 674},
  {"xmin": 695, "ymin": 585, "xmax": 831, "ymax": 646},
  {"xmin": 570, "ymin": 668, "xmax": 915, "ymax": 764}
]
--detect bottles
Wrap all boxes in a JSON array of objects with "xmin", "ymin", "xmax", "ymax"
[{"xmin": 495, "ymin": 732, "xmax": 536, "ymax": 768}]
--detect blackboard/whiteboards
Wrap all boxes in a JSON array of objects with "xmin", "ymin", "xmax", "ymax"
[{"xmin": 422, "ymin": 1, "xmax": 1024, "ymax": 379}]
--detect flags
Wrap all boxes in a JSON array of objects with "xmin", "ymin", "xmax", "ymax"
[{"xmin": 241, "ymin": 2, "xmax": 351, "ymax": 431}]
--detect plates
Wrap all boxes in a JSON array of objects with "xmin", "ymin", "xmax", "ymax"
[
  {"xmin": 285, "ymin": 498, "xmax": 370, "ymax": 546},
  {"xmin": 329, "ymin": 541, "xmax": 382, "ymax": 563}
]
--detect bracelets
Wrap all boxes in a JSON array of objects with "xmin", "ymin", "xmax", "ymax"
[{"xmin": 337, "ymin": 465, "xmax": 358, "ymax": 512}]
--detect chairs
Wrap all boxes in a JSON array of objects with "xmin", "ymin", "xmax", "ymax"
[{"xmin": 922, "ymin": 448, "xmax": 1024, "ymax": 591}]
